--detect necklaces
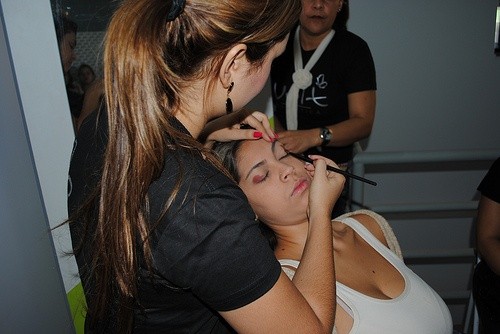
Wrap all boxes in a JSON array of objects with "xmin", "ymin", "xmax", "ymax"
[{"xmin": 286, "ymin": 24, "xmax": 335, "ymax": 130}]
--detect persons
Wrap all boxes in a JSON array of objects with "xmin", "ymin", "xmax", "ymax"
[
  {"xmin": 270, "ymin": 0, "xmax": 377, "ymax": 219},
  {"xmin": 55, "ymin": 18, "xmax": 105, "ymax": 135},
  {"xmin": 210, "ymin": 126, "xmax": 453, "ymax": 334},
  {"xmin": 67, "ymin": 0, "xmax": 346, "ymax": 334},
  {"xmin": 472, "ymin": 156, "xmax": 500, "ymax": 334}
]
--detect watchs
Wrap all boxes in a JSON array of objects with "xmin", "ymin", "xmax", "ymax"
[{"xmin": 320, "ymin": 126, "xmax": 333, "ymax": 147}]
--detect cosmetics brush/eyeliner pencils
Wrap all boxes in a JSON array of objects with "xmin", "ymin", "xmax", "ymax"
[{"xmin": 284, "ymin": 149, "xmax": 377, "ymax": 186}]
